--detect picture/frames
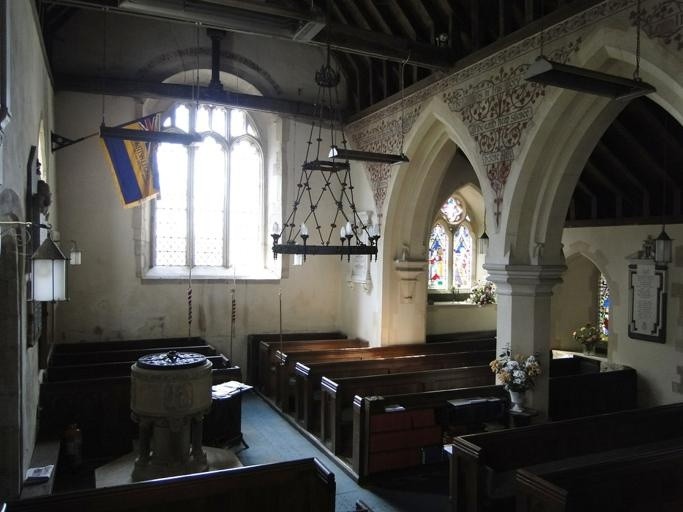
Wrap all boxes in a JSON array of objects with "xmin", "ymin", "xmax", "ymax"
[
  {"xmin": 348, "ymin": 210, "xmax": 371, "ymax": 283},
  {"xmin": 625, "ymin": 248, "xmax": 667, "ymax": 344},
  {"xmin": 27, "ymin": 145, "xmax": 42, "ymax": 345}
]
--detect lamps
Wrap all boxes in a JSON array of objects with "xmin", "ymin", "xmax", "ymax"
[
  {"xmin": 328, "ymin": 52, "xmax": 409, "ymax": 165},
  {"xmin": 479, "ymin": 232, "xmax": 489, "ymax": 254},
  {"xmin": 525, "ymin": 1, "xmax": 656, "ymax": 101},
  {"xmin": 69, "ymin": 240, "xmax": 81, "ymax": 265},
  {"xmin": 99, "ymin": 10, "xmax": 201, "ymax": 145},
  {"xmin": 654, "ymin": 223, "xmax": 674, "ymax": 263},
  {"xmin": 28, "ymin": 223, "xmax": 70, "ymax": 303},
  {"xmin": 116, "ymin": 0, "xmax": 326, "ymax": 43}
]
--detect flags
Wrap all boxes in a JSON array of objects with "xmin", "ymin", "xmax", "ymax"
[{"xmin": 99, "ymin": 112, "xmax": 160, "ymax": 208}]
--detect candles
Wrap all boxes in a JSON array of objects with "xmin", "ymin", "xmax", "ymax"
[
  {"xmin": 340, "ymin": 226, "xmax": 346, "ymax": 238},
  {"xmin": 346, "ymin": 221, "xmax": 353, "ymax": 236},
  {"xmin": 273, "ymin": 222, "xmax": 279, "ymax": 235},
  {"xmin": 368, "ymin": 227, "xmax": 373, "ymax": 238},
  {"xmin": 374, "ymin": 224, "xmax": 378, "ymax": 236},
  {"xmin": 301, "ymin": 223, "xmax": 308, "ymax": 235}
]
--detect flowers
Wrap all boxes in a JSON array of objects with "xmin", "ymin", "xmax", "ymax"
[
  {"xmin": 467, "ymin": 280, "xmax": 496, "ymax": 304},
  {"xmin": 573, "ymin": 322, "xmax": 601, "ymax": 346}
]
